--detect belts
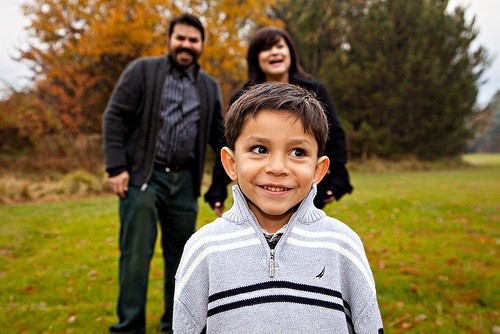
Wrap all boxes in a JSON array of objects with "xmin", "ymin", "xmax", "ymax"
[{"xmin": 155, "ymin": 160, "xmax": 191, "ymax": 173}]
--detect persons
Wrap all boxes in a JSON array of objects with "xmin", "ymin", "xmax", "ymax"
[
  {"xmin": 101, "ymin": 15, "xmax": 234, "ymax": 333},
  {"xmin": 206, "ymin": 25, "xmax": 353, "ymax": 216},
  {"xmin": 171, "ymin": 80, "xmax": 386, "ymax": 334}
]
